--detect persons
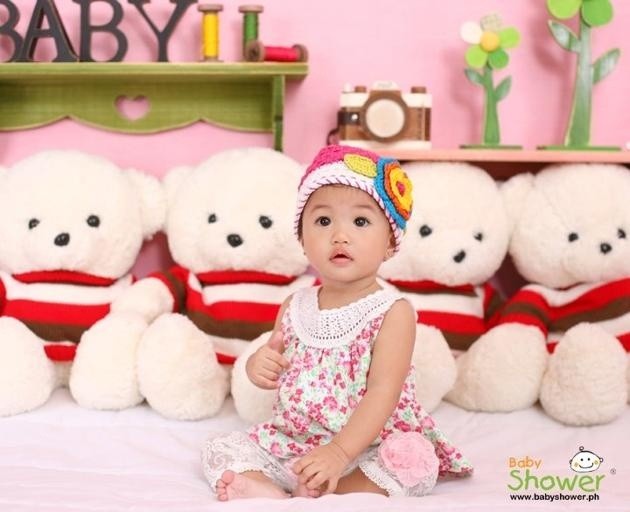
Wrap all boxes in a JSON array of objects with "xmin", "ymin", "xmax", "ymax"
[
  {"xmin": 190, "ymin": 144, "xmax": 479, "ymax": 507},
  {"xmin": 0, "ymin": 151, "xmax": 172, "ymax": 413}
]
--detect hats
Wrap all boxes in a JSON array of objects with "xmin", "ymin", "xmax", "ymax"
[{"xmin": 292, "ymin": 143, "xmax": 415, "ymax": 261}]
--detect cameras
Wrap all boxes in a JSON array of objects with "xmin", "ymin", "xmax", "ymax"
[{"xmin": 337, "ymin": 81, "xmax": 432, "ymax": 150}]
